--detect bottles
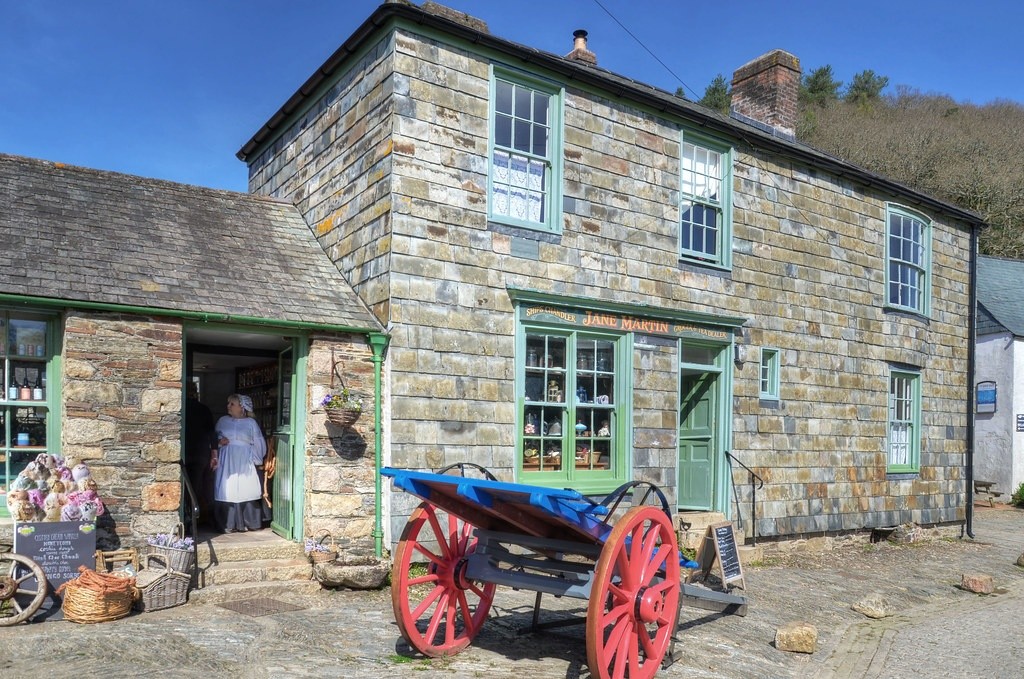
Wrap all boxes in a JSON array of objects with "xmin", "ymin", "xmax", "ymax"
[
  {"xmin": 528, "ymin": 347, "xmax": 609, "ymax": 371},
  {"xmin": 576, "ymin": 420, "xmax": 587, "ymax": 437},
  {"xmin": 533, "ymin": 414, "xmax": 539, "ymax": 434},
  {"xmin": 577, "ymin": 386, "xmax": 588, "ymax": 403},
  {"xmin": 8, "ymin": 375, "xmax": 42, "ymax": 401}
]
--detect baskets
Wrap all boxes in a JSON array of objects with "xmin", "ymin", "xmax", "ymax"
[
  {"xmin": 61, "ymin": 579, "xmax": 138, "ymax": 623},
  {"xmin": 134, "ymin": 553, "xmax": 192, "ymax": 612},
  {"xmin": 311, "ymin": 528, "xmax": 334, "ymax": 563},
  {"xmin": 147, "ymin": 522, "xmax": 196, "ymax": 574}
]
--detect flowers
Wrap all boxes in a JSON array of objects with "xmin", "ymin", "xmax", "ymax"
[
  {"xmin": 148, "ymin": 532, "xmax": 195, "ymax": 552},
  {"xmin": 323, "ymin": 393, "xmax": 361, "ymax": 410},
  {"xmin": 305, "ymin": 538, "xmax": 329, "ymax": 555}
]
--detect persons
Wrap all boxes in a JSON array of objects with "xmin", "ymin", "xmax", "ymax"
[
  {"xmin": 215, "ymin": 393, "xmax": 267, "ymax": 535},
  {"xmin": 184, "ymin": 382, "xmax": 219, "ymax": 532}
]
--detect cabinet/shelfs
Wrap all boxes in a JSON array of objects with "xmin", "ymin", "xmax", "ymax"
[
  {"xmin": 236, "ymin": 360, "xmax": 279, "ymax": 433},
  {"xmin": 523, "ymin": 335, "xmax": 614, "ymax": 471}
]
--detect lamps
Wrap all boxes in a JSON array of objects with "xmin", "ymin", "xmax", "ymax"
[{"xmin": 735, "ymin": 343, "xmax": 748, "ymax": 363}]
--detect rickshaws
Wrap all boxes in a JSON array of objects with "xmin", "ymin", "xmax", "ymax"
[{"xmin": 378, "ymin": 460, "xmax": 750, "ymax": 679}]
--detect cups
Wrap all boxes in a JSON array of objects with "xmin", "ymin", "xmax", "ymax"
[{"xmin": 18, "ymin": 433, "xmax": 29, "ymax": 446}]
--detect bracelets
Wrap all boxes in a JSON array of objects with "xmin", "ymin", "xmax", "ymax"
[{"xmin": 211, "ymin": 457, "xmax": 218, "ymax": 460}]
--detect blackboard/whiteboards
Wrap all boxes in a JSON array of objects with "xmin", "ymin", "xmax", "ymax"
[
  {"xmin": 12, "ymin": 519, "xmax": 97, "ymax": 624},
  {"xmin": 711, "ymin": 521, "xmax": 743, "ymax": 583}
]
